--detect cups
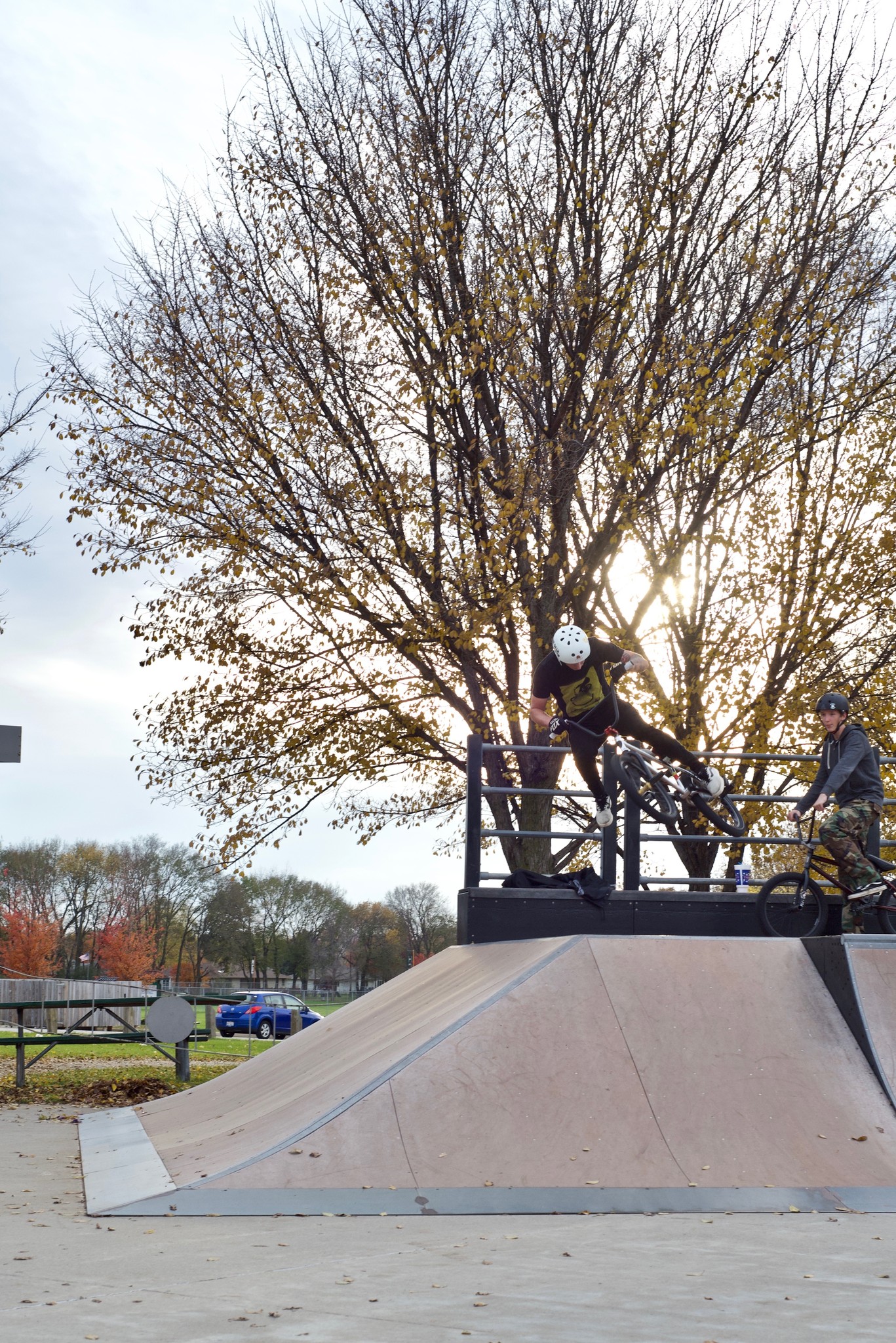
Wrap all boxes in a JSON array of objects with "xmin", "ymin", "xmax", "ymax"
[{"xmin": 734, "ymin": 865, "xmax": 750, "ymax": 893}]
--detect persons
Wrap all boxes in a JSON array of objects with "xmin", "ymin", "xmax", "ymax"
[
  {"xmin": 788, "ymin": 691, "xmax": 884, "ymax": 937},
  {"xmin": 529, "ymin": 626, "xmax": 726, "ymax": 827}
]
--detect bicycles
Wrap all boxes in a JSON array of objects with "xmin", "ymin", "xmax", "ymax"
[
  {"xmin": 548, "ymin": 674, "xmax": 748, "ymax": 836},
  {"xmin": 755, "ymin": 795, "xmax": 896, "ymax": 934}
]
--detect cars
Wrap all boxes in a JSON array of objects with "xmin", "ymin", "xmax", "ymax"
[{"xmin": 216, "ymin": 989, "xmax": 324, "ymax": 1041}]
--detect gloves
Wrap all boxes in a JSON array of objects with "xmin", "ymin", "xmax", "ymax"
[
  {"xmin": 609, "ymin": 662, "xmax": 626, "ymax": 683},
  {"xmin": 548, "ymin": 715, "xmax": 568, "ymax": 736}
]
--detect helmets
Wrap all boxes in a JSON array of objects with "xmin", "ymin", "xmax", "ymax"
[
  {"xmin": 815, "ymin": 690, "xmax": 851, "ymax": 714},
  {"xmin": 552, "ymin": 624, "xmax": 590, "ymax": 664}
]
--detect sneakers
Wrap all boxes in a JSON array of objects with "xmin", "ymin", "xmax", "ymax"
[
  {"xmin": 596, "ymin": 795, "xmax": 613, "ymax": 827},
  {"xmin": 848, "ymin": 877, "xmax": 888, "ymax": 899},
  {"xmin": 698, "ymin": 764, "xmax": 724, "ymax": 797}
]
